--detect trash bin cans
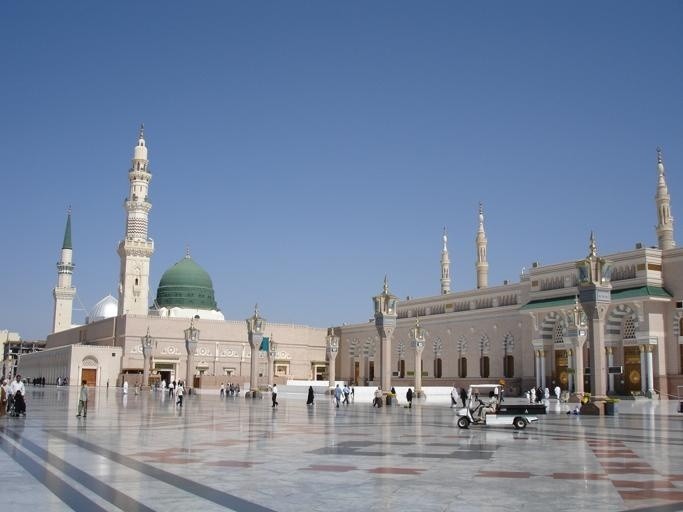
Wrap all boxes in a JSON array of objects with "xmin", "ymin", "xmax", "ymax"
[
  {"xmin": 605, "ymin": 398, "xmax": 619, "ymax": 416},
  {"xmin": 386, "ymin": 393, "xmax": 397, "ymax": 405}
]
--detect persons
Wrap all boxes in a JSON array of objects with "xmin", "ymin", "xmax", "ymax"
[
  {"xmin": 525, "ymin": 379, "xmax": 561, "ymax": 404},
  {"xmin": 372, "ymin": 386, "xmax": 397, "ymax": 408},
  {"xmin": 123, "ymin": 379, "xmax": 143, "ymax": 395},
  {"xmin": 406, "ymin": 388, "xmax": 412, "ymax": 408},
  {"xmin": 494, "ymin": 385, "xmax": 499, "ymax": 400},
  {"xmin": 306, "ymin": 386, "xmax": 315, "ymax": 405},
  {"xmin": 499, "ymin": 384, "xmax": 504, "ymax": 403},
  {"xmin": 107, "ymin": 378, "xmax": 109, "ymax": 388},
  {"xmin": 150, "ymin": 377, "xmax": 184, "ymax": 408},
  {"xmin": 334, "ymin": 384, "xmax": 354, "ymax": 408},
  {"xmin": 219, "ymin": 382, "xmax": 240, "ymax": 400},
  {"xmin": 32, "ymin": 376, "xmax": 46, "ymax": 387},
  {"xmin": 75, "ymin": 379, "xmax": 89, "ymax": 418},
  {"xmin": 56, "ymin": 375, "xmax": 69, "ymax": 387},
  {"xmin": 460, "ymin": 388, "xmax": 467, "ymax": 408},
  {"xmin": 450, "ymin": 388, "xmax": 458, "ymax": 408},
  {"xmin": 0, "ymin": 374, "xmax": 26, "ymax": 418},
  {"xmin": 271, "ymin": 384, "xmax": 278, "ymax": 407},
  {"xmin": 474, "ymin": 391, "xmax": 496, "ymax": 424}
]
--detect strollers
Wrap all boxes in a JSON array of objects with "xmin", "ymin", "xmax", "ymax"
[{"xmin": 11, "ymin": 390, "xmax": 26, "ymax": 418}]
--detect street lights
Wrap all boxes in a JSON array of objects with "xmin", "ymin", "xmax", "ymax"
[
  {"xmin": 246, "ymin": 303, "xmax": 264, "ymax": 391},
  {"xmin": 142, "ymin": 327, "xmax": 153, "ymax": 386},
  {"xmin": 266, "ymin": 334, "xmax": 276, "ymax": 385},
  {"xmin": 373, "ymin": 276, "xmax": 401, "ymax": 394},
  {"xmin": 408, "ymin": 317, "xmax": 425, "ymax": 392},
  {"xmin": 575, "ymin": 232, "xmax": 610, "ymax": 400},
  {"xmin": 564, "ymin": 293, "xmax": 587, "ymax": 393},
  {"xmin": 183, "ymin": 317, "xmax": 199, "ymax": 388},
  {"xmin": 326, "ymin": 328, "xmax": 339, "ymax": 387}
]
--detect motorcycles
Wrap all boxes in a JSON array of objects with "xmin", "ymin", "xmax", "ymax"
[{"xmin": 456, "ymin": 383, "xmax": 546, "ymax": 430}]
